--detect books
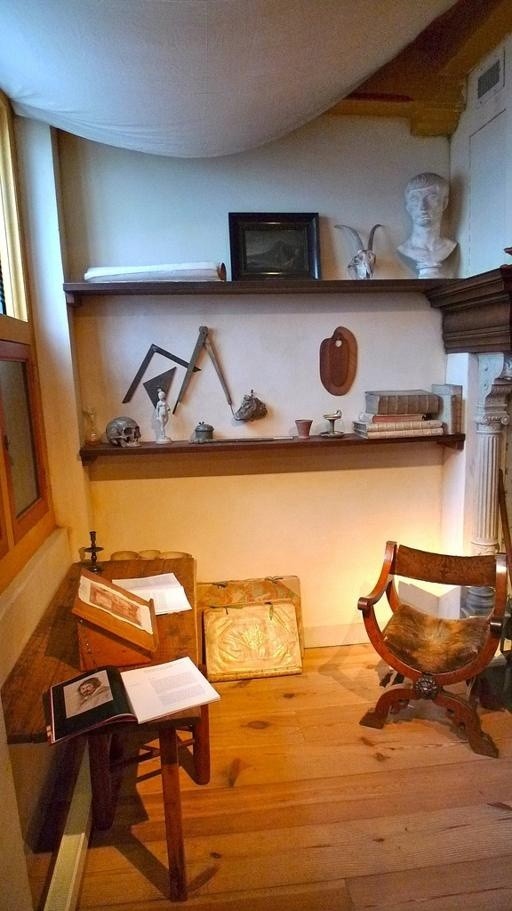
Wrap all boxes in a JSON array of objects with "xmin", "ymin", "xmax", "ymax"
[
  {"xmin": 431, "ymin": 383, "xmax": 463, "ymax": 432},
  {"xmin": 438, "ymin": 394, "xmax": 457, "ymax": 436},
  {"xmin": 352, "ymin": 419, "xmax": 444, "ymax": 432},
  {"xmin": 364, "ymin": 388, "xmax": 439, "ymax": 417},
  {"xmin": 357, "ymin": 413, "xmax": 428, "ymax": 423},
  {"xmin": 45, "ymin": 656, "xmax": 222, "ymax": 748},
  {"xmin": 352, "ymin": 428, "xmax": 445, "ymax": 440}
]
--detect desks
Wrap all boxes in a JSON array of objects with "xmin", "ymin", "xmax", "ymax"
[{"xmin": 0, "ymin": 556, "xmax": 210, "ymax": 911}]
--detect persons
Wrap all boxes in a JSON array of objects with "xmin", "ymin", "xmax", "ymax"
[{"xmin": 397, "ymin": 170, "xmax": 459, "ymax": 264}]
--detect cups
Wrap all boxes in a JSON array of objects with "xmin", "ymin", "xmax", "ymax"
[{"xmin": 295, "ymin": 419, "xmax": 313, "ymax": 439}]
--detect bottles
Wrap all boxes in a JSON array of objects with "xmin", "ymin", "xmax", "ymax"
[{"xmin": 84, "ymin": 407, "xmax": 102, "ymax": 446}]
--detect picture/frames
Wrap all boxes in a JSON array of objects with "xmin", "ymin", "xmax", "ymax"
[
  {"xmin": 228, "ymin": 211, "xmax": 323, "ymax": 284},
  {"xmin": 72, "ymin": 566, "xmax": 160, "ymax": 654}
]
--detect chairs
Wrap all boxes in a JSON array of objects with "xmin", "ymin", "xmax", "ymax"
[{"xmin": 356, "ymin": 535, "xmax": 508, "ymax": 758}]
